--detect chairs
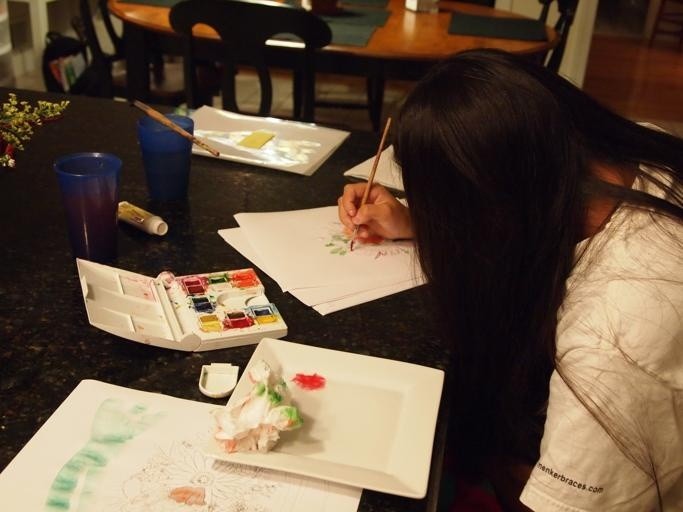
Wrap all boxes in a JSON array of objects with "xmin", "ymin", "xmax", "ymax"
[
  {"xmin": 535, "ymin": 0, "xmax": 581, "ymax": 72},
  {"xmin": 295, "ymin": 68, "xmax": 387, "ymax": 134},
  {"xmin": 169, "ymin": 2, "xmax": 333, "ymax": 124},
  {"xmin": 73, "ymin": 0, "xmax": 164, "ymax": 103}
]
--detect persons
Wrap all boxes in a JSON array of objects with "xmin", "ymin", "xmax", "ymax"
[{"xmin": 334, "ymin": 44, "xmax": 681, "ymax": 512}]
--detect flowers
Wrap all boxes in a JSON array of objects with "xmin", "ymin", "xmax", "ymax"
[{"xmin": 0, "ymin": 89, "xmax": 71, "ymax": 169}]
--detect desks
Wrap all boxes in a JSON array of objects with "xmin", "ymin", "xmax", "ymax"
[
  {"xmin": 108, "ymin": 1, "xmax": 563, "ymax": 132},
  {"xmin": 0, "ymin": 84, "xmax": 451, "ymax": 512}
]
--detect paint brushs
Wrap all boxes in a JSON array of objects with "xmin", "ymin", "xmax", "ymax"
[
  {"xmin": 127, "ymin": 98, "xmax": 220, "ymax": 158},
  {"xmin": 351, "ymin": 117, "xmax": 393, "ymax": 251}
]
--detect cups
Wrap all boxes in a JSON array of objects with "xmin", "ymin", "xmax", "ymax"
[
  {"xmin": 55, "ymin": 154, "xmax": 123, "ymax": 265},
  {"xmin": 138, "ymin": 114, "xmax": 195, "ymax": 230}
]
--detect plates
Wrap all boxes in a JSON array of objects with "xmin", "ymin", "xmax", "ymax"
[
  {"xmin": 343, "ymin": 142, "xmax": 405, "ymax": 195},
  {"xmin": 206, "ymin": 337, "xmax": 448, "ymax": 500}
]
